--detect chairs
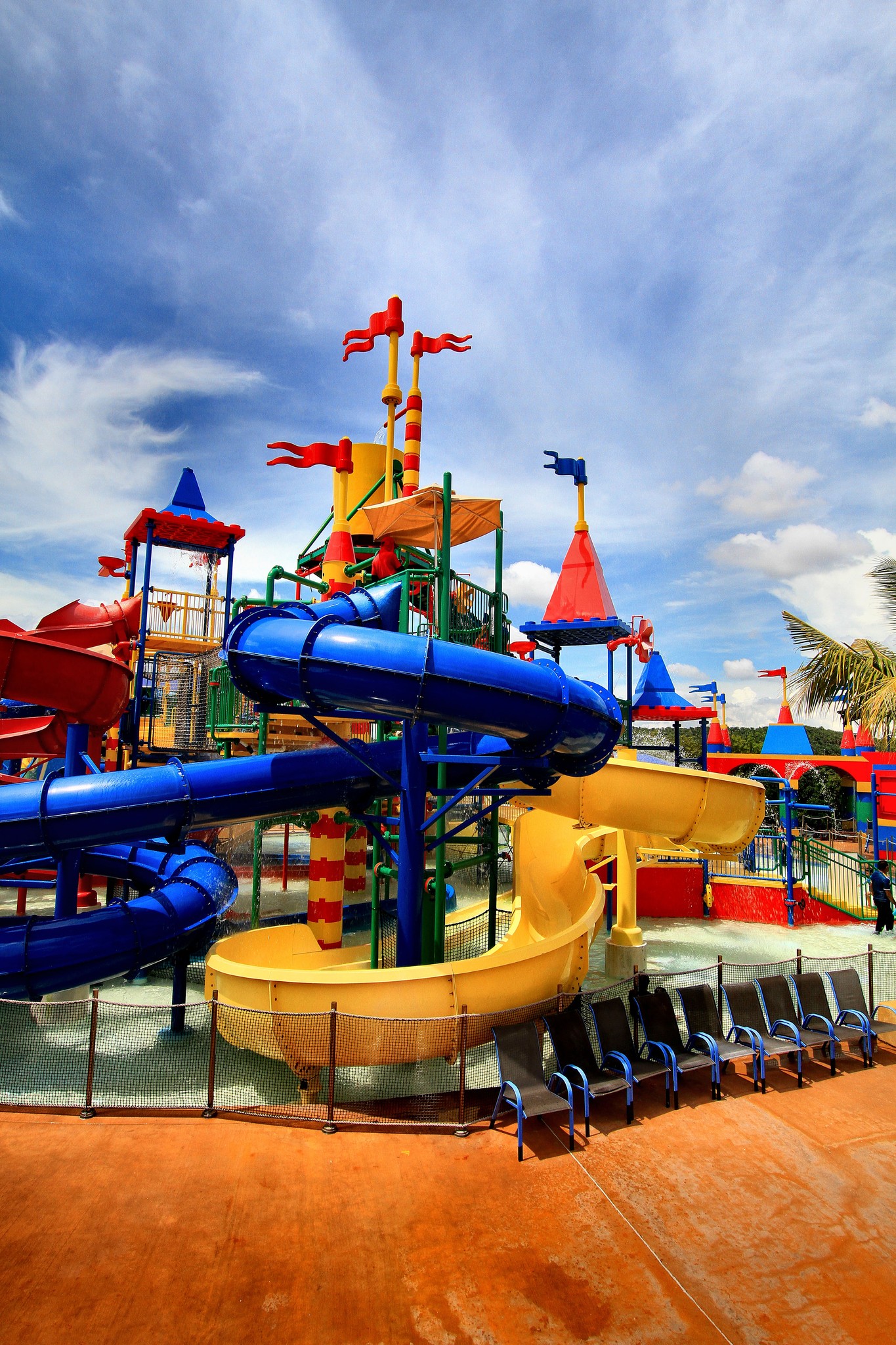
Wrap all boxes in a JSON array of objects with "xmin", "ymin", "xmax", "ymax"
[
  {"xmin": 789, "ymin": 970, "xmax": 868, "ymax": 1075},
  {"xmin": 632, "ymin": 994, "xmax": 716, "ymax": 1111},
  {"xmin": 718, "ymin": 981, "xmax": 801, "ymax": 1094},
  {"xmin": 587, "ymin": 996, "xmax": 672, "ymax": 1122},
  {"xmin": 542, "ymin": 1008, "xmax": 632, "ymax": 1137},
  {"xmin": 753, "ymin": 974, "xmax": 835, "ymax": 1086},
  {"xmin": 676, "ymin": 984, "xmax": 760, "ymax": 1101},
  {"xmin": 825, "ymin": 967, "xmax": 896, "ymax": 1067},
  {"xmin": 488, "ymin": 1020, "xmax": 576, "ymax": 1163}
]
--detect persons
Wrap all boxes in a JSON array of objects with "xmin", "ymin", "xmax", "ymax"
[
  {"xmin": 856, "ymin": 867, "xmax": 874, "ymax": 909},
  {"xmin": 655, "ymin": 987, "xmax": 667, "ymax": 994},
  {"xmin": 475, "ymin": 592, "xmax": 509, "ymax": 654},
  {"xmin": 870, "ymin": 860, "xmax": 896, "ymax": 935},
  {"xmin": 371, "ymin": 537, "xmax": 405, "ymax": 582},
  {"xmin": 628, "ymin": 975, "xmax": 654, "ymax": 1024},
  {"xmin": 776, "ymin": 826, "xmax": 788, "ymax": 867}
]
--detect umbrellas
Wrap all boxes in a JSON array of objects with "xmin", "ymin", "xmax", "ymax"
[{"xmin": 358, "ymin": 482, "xmax": 508, "ymax": 640}]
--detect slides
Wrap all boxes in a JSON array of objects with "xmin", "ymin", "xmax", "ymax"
[{"xmin": 1, "ymin": 577, "xmax": 773, "ymax": 1076}]
